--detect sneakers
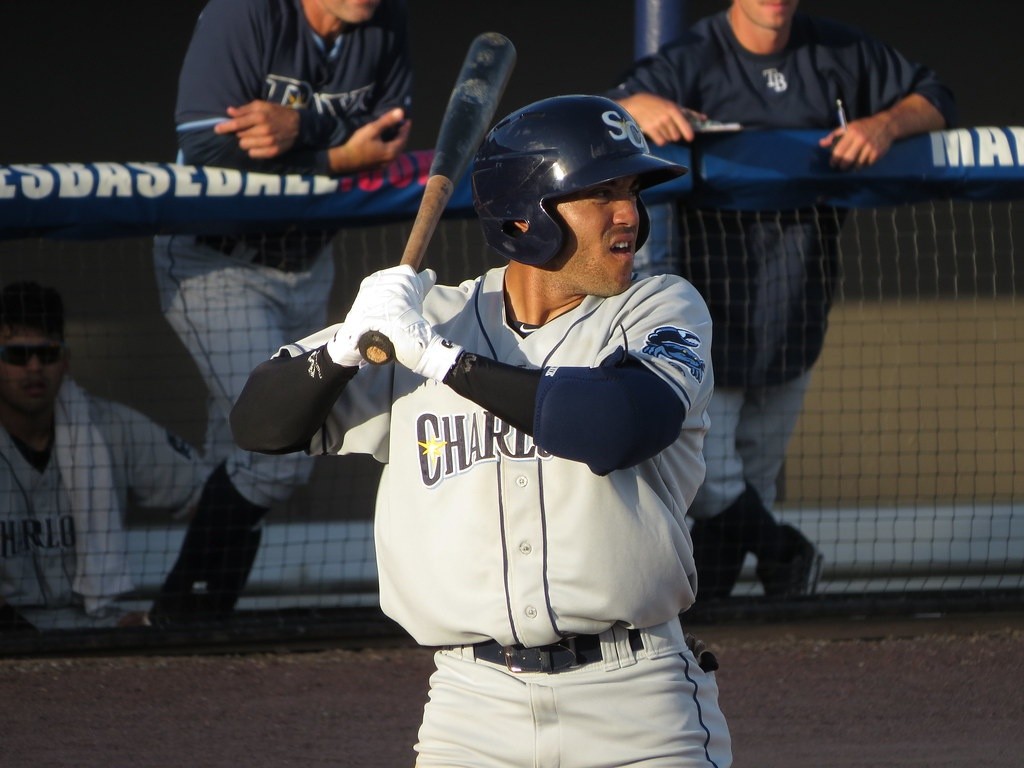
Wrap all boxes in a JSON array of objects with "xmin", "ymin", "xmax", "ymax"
[{"xmin": 757, "ymin": 528, "xmax": 824, "ymax": 603}]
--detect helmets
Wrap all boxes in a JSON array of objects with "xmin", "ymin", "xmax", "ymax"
[{"xmin": 471, "ymin": 96, "xmax": 691, "ymax": 268}]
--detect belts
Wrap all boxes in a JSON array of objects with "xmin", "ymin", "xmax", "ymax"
[{"xmin": 474, "ymin": 629, "xmax": 644, "ymax": 673}]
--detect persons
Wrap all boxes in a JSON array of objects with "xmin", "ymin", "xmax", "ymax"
[
  {"xmin": 229, "ymin": 92, "xmax": 740, "ymax": 768},
  {"xmin": 147, "ymin": 0, "xmax": 424, "ymax": 632},
  {"xmin": 0, "ymin": 280, "xmax": 209, "ymax": 630},
  {"xmin": 604, "ymin": 2, "xmax": 968, "ymax": 599}
]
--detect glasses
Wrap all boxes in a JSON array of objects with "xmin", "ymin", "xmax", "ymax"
[{"xmin": 1, "ymin": 344, "xmax": 62, "ymax": 366}]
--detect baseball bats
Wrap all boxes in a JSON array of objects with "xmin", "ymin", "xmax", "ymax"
[{"xmin": 356, "ymin": 29, "xmax": 521, "ymax": 368}]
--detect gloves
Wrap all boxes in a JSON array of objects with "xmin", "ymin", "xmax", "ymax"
[{"xmin": 327, "ymin": 264, "xmax": 464, "ymax": 381}]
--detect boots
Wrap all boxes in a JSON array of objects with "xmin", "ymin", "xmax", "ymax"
[{"xmin": 148, "ymin": 460, "xmax": 271, "ymax": 635}]
